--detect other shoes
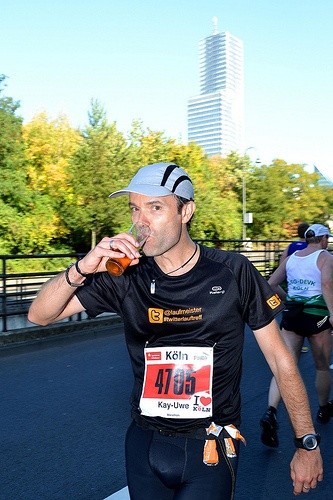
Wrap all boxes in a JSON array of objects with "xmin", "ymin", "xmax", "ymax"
[
  {"xmin": 317, "ymin": 399, "xmax": 333, "ymax": 426},
  {"xmin": 259, "ymin": 409, "xmax": 279, "ymax": 447}
]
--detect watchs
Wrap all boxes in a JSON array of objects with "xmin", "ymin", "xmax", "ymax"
[{"xmin": 295, "ymin": 434, "xmax": 321, "ymax": 451}]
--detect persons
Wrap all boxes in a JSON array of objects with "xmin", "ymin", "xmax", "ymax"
[
  {"xmin": 28, "ymin": 161, "xmax": 323, "ymax": 500},
  {"xmin": 260, "ymin": 224, "xmax": 333, "ymax": 447},
  {"xmin": 280, "ymin": 224, "xmax": 310, "ymax": 353}
]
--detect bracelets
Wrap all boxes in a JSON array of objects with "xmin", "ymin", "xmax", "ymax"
[
  {"xmin": 76, "ymin": 259, "xmax": 94, "ymax": 278},
  {"xmin": 66, "ymin": 263, "xmax": 88, "ymax": 287}
]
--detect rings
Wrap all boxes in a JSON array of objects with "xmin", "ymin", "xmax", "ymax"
[
  {"xmin": 110, "ymin": 240, "xmax": 115, "ymax": 251},
  {"xmin": 303, "ymin": 486, "xmax": 311, "ymax": 490}
]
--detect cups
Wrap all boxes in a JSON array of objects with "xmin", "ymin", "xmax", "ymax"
[{"xmin": 106, "ymin": 221, "xmax": 151, "ymax": 277}]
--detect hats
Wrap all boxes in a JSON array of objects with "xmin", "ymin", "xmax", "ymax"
[
  {"xmin": 304, "ymin": 223, "xmax": 333, "ymax": 239},
  {"xmin": 108, "ymin": 162, "xmax": 195, "ymax": 201}
]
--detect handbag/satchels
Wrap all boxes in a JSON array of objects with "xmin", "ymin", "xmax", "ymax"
[{"xmin": 282, "ymin": 301, "xmax": 303, "ymax": 319}]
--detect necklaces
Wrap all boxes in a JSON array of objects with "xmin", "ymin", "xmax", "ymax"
[{"xmin": 150, "ymin": 244, "xmax": 198, "ymax": 294}]
